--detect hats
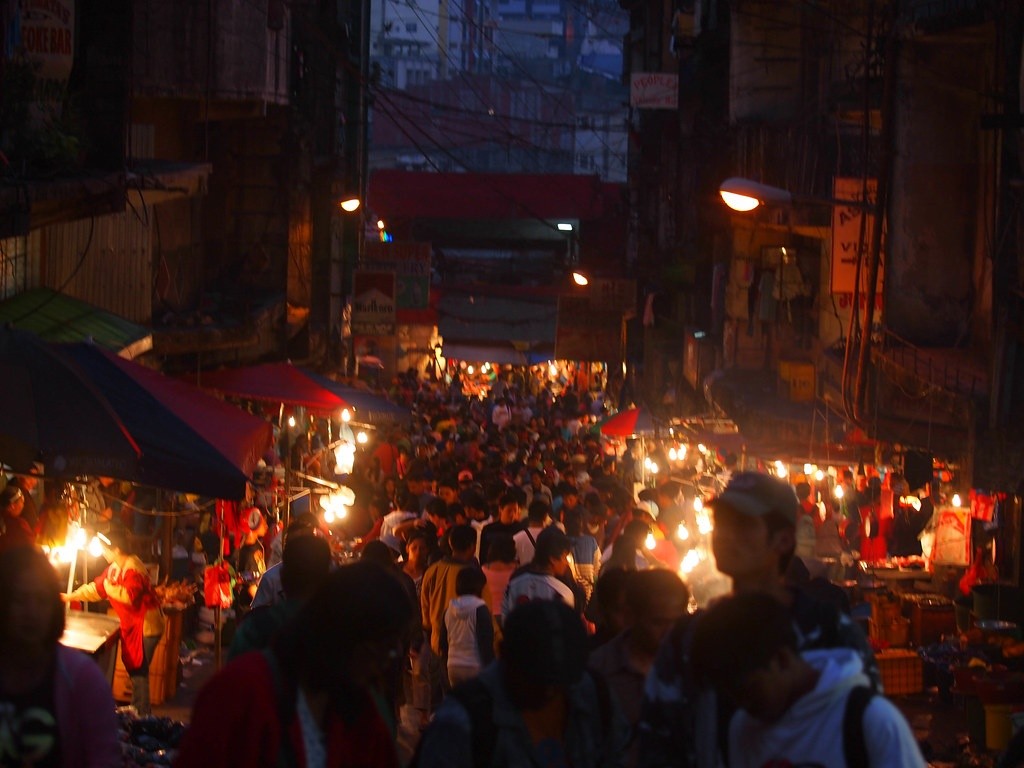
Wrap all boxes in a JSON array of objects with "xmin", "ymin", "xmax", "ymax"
[{"xmin": 706, "ymin": 472, "xmax": 795, "ymax": 525}]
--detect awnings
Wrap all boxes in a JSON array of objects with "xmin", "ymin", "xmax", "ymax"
[{"xmin": 0, "ymin": 285, "xmax": 153, "ymax": 363}]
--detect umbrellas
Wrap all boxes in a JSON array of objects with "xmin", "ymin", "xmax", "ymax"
[
  {"xmin": 171, "ymin": 359, "xmax": 412, "ymax": 534},
  {"xmin": 587, "ymin": 407, "xmax": 677, "ymax": 442},
  {"xmin": 0, "ymin": 328, "xmax": 151, "ymax": 486},
  {"xmin": 86, "ymin": 344, "xmax": 278, "ymax": 476},
  {"xmin": 40, "ymin": 341, "xmax": 248, "ymax": 505}
]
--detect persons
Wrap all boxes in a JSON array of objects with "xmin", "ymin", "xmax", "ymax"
[{"xmin": 0, "ymin": 359, "xmax": 1023, "ymax": 768}]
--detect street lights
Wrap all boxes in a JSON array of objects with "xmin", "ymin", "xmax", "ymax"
[{"xmin": 721, "ymin": 176, "xmax": 884, "ymax": 432}]
